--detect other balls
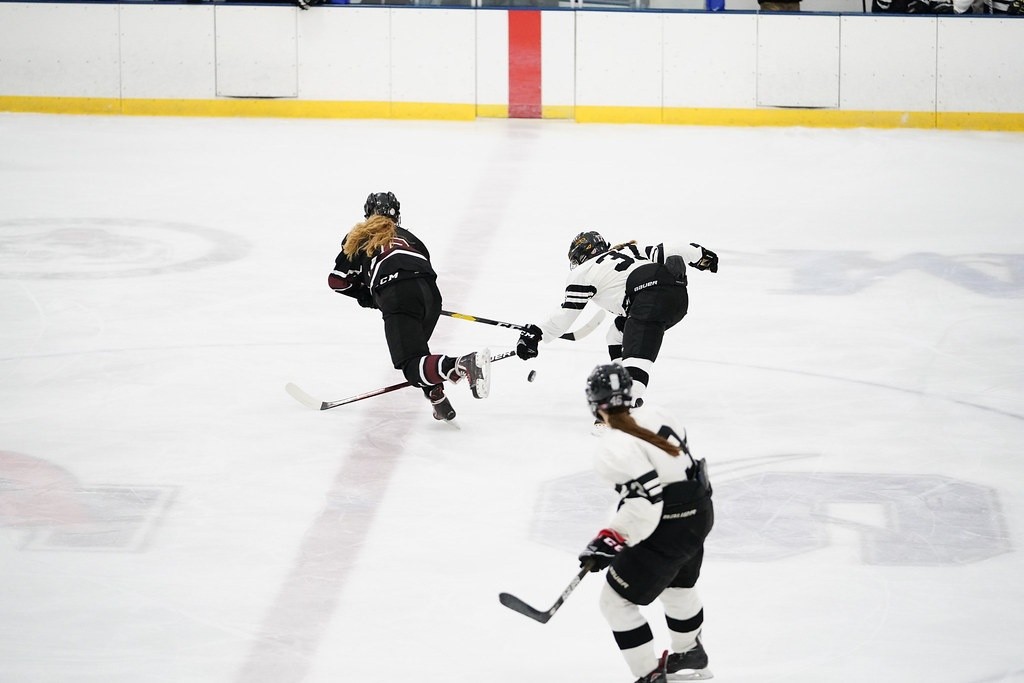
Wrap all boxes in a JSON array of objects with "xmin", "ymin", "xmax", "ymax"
[{"xmin": 527, "ymin": 370, "xmax": 536, "ymax": 382}]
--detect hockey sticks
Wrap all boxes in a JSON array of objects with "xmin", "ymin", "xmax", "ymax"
[
  {"xmin": 439, "ymin": 309, "xmax": 607, "ymax": 342},
  {"xmin": 498, "ymin": 558, "xmax": 597, "ymax": 624},
  {"xmin": 284, "ymin": 348, "xmax": 516, "ymax": 411}
]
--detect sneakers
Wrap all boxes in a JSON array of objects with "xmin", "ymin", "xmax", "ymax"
[
  {"xmin": 634, "ymin": 649, "xmax": 667, "ymax": 683},
  {"xmin": 432, "ymin": 397, "xmax": 462, "ymax": 431},
  {"xmin": 458, "ymin": 347, "xmax": 490, "ymax": 399},
  {"xmin": 659, "ymin": 628, "xmax": 713, "ymax": 679}
]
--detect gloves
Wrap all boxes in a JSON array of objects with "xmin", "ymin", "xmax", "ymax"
[
  {"xmin": 579, "ymin": 532, "xmax": 629, "ymax": 572},
  {"xmin": 517, "ymin": 324, "xmax": 543, "ymax": 360},
  {"xmin": 360, "ymin": 287, "xmax": 382, "ymax": 311},
  {"xmin": 689, "ymin": 242, "xmax": 718, "ymax": 274}
]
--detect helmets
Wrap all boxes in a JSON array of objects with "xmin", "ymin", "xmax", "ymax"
[
  {"xmin": 585, "ymin": 363, "xmax": 633, "ymax": 420},
  {"xmin": 364, "ymin": 191, "xmax": 400, "ymax": 219},
  {"xmin": 568, "ymin": 231, "xmax": 611, "ymax": 271}
]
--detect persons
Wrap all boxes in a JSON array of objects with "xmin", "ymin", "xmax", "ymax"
[
  {"xmin": 328, "ymin": 192, "xmax": 484, "ymax": 421},
  {"xmin": 578, "ymin": 363, "xmax": 714, "ymax": 683},
  {"xmin": 516, "ymin": 231, "xmax": 718, "ymax": 425}
]
